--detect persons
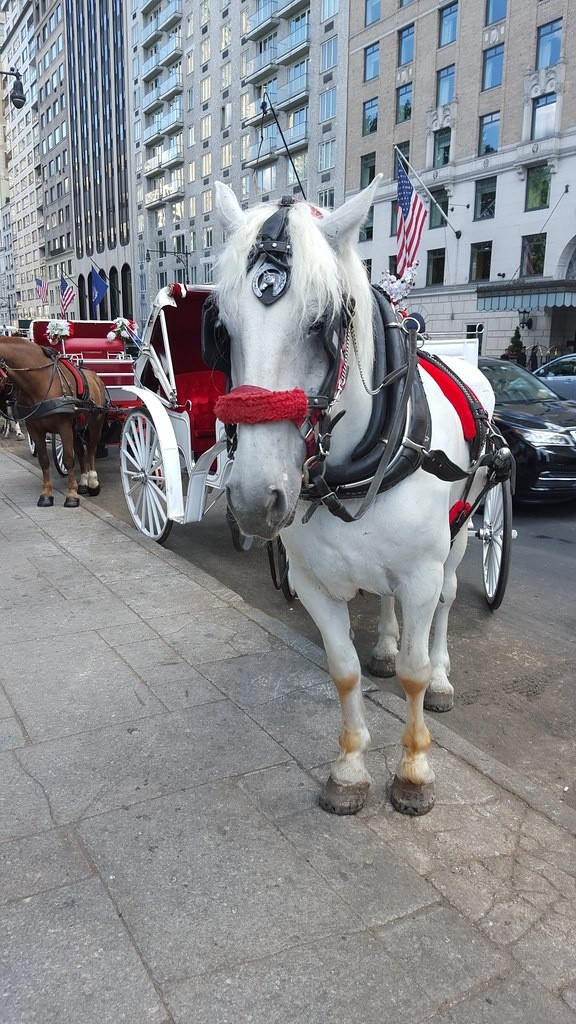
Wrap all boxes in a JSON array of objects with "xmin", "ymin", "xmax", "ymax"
[
  {"xmin": 516, "ymin": 345, "xmax": 527, "ymax": 367},
  {"xmin": 18, "ymin": 330, "xmax": 23, "ymax": 337},
  {"xmin": 500, "ymin": 349, "xmax": 509, "ymax": 361},
  {"xmin": 530, "ymin": 346, "xmax": 539, "ymax": 371}
]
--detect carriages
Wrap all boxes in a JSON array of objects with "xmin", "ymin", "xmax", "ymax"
[
  {"xmin": 0, "ymin": 317, "xmax": 143, "ymax": 507},
  {"xmin": 117, "ymin": 170, "xmax": 519, "ymax": 818}
]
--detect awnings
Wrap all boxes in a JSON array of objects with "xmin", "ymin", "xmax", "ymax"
[{"xmin": 476, "ymin": 279, "xmax": 576, "ymax": 311}]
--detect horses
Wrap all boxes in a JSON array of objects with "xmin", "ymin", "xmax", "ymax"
[
  {"xmin": 206, "ymin": 170, "xmax": 499, "ymax": 817},
  {"xmin": 0, "ymin": 335, "xmax": 106, "ymax": 508}
]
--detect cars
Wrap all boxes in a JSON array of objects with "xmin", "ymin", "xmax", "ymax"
[
  {"xmin": 507, "ymin": 352, "xmax": 576, "ymax": 402},
  {"xmin": 478, "ymin": 354, "xmax": 576, "ymax": 508}
]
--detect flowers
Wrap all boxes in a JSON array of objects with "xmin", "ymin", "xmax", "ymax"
[
  {"xmin": 44, "ymin": 319, "xmax": 75, "ymax": 346},
  {"xmin": 106, "ymin": 317, "xmax": 140, "ymax": 343}
]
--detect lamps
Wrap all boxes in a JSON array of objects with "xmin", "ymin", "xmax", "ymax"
[{"xmin": 518, "ymin": 309, "xmax": 532, "ymax": 330}]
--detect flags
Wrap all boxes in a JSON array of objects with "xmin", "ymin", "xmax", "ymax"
[
  {"xmin": 35, "ymin": 279, "xmax": 48, "ymax": 302},
  {"xmin": 60, "ymin": 275, "xmax": 75, "ymax": 317},
  {"xmin": 397, "ymin": 162, "xmax": 427, "ymax": 276},
  {"xmin": 92, "ymin": 265, "xmax": 108, "ymax": 312}
]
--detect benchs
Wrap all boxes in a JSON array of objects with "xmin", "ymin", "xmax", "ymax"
[
  {"xmin": 159, "ymin": 370, "xmax": 227, "ymax": 452},
  {"xmin": 58, "ymin": 336, "xmax": 136, "ymax": 386}
]
